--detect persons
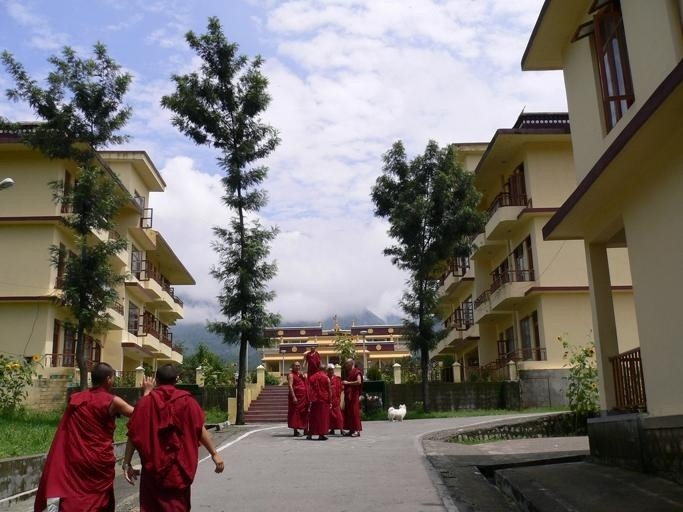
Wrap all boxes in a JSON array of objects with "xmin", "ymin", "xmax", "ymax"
[
  {"xmin": 33, "ymin": 360, "xmax": 156, "ymax": 511},
  {"xmin": 341, "ymin": 357, "xmax": 364, "ymax": 438},
  {"xmin": 119, "ymin": 362, "xmax": 225, "ymax": 512},
  {"xmin": 287, "ymin": 360, "xmax": 309, "ymax": 437},
  {"xmin": 305, "ymin": 364, "xmax": 332, "ymax": 441},
  {"xmin": 324, "ymin": 362, "xmax": 346, "ymax": 437},
  {"xmin": 301, "ymin": 346, "xmax": 321, "ymax": 380}
]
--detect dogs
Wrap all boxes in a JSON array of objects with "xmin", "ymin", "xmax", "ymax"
[{"xmin": 387, "ymin": 403, "xmax": 407, "ymax": 422}]
